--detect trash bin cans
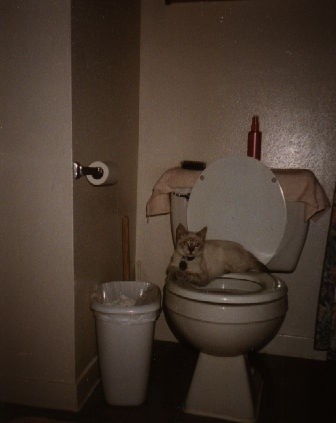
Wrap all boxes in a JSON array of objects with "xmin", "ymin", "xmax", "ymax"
[{"xmin": 92, "ymin": 280, "xmax": 162, "ymax": 406}]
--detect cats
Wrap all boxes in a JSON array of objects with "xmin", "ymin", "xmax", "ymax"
[{"xmin": 165, "ymin": 222, "xmax": 270, "ymax": 289}]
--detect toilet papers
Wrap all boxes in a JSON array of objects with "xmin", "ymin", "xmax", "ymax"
[{"xmin": 85, "ymin": 160, "xmax": 120, "ymax": 186}]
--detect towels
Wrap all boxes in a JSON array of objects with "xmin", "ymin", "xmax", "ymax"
[{"xmin": 146, "ymin": 168, "xmax": 331, "ymax": 222}]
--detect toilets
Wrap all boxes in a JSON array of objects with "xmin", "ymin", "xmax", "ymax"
[{"xmin": 162, "ymin": 156, "xmax": 310, "ymax": 423}]
